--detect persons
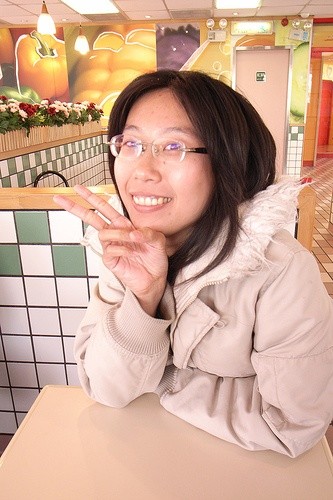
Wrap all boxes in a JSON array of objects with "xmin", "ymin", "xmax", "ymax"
[{"xmin": 52, "ymin": 70, "xmax": 333, "ymax": 458}]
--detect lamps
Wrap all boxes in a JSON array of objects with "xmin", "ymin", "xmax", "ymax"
[
  {"xmin": 289, "ymin": 18, "xmax": 312, "ymax": 42},
  {"xmin": 206, "ymin": 19, "xmax": 227, "ymax": 43},
  {"xmin": 74, "ymin": 21, "xmax": 89, "ymax": 53},
  {"xmin": 37, "ymin": 0, "xmax": 56, "ymax": 36}
]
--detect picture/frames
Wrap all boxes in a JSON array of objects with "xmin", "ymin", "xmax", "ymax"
[{"xmin": 0, "ymin": 121, "xmax": 101, "ymax": 153}]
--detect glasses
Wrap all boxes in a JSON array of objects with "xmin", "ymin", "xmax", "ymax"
[{"xmin": 105, "ymin": 134, "xmax": 209, "ymax": 162}]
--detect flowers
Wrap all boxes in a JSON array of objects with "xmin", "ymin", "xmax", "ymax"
[{"xmin": 0, "ymin": 96, "xmax": 104, "ymax": 138}]
